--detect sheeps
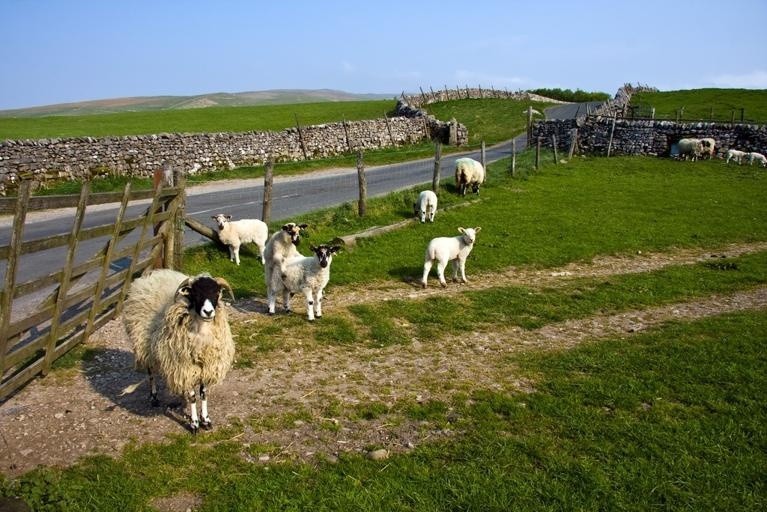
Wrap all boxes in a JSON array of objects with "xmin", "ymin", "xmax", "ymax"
[
  {"xmin": 421, "ymin": 226, "xmax": 482, "ymax": 289},
  {"xmin": 263, "ymin": 222, "xmax": 342, "ymax": 323},
  {"xmin": 455, "ymin": 158, "xmax": 484, "ymax": 198},
  {"xmin": 747, "ymin": 152, "xmax": 767, "ymax": 168},
  {"xmin": 121, "ymin": 267, "xmax": 236, "ymax": 436},
  {"xmin": 726, "ymin": 150, "xmax": 750, "ymax": 165},
  {"xmin": 678, "ymin": 138, "xmax": 716, "ymax": 162},
  {"xmin": 412, "ymin": 190, "xmax": 438, "ymax": 224},
  {"xmin": 210, "ymin": 213, "xmax": 269, "ymax": 266}
]
